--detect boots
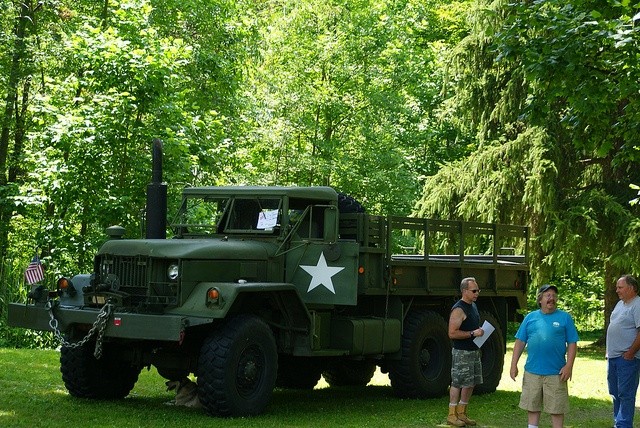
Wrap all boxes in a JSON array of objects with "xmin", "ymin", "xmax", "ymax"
[
  {"xmin": 458, "ymin": 403, "xmax": 476, "ymax": 425},
  {"xmin": 446, "ymin": 404, "xmax": 465, "ymax": 427}
]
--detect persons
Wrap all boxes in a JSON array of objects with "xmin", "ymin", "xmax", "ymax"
[
  {"xmin": 605, "ymin": 276, "xmax": 640, "ymax": 428},
  {"xmin": 510, "ymin": 282, "xmax": 579, "ymax": 428},
  {"xmin": 447, "ymin": 277, "xmax": 484, "ymax": 426}
]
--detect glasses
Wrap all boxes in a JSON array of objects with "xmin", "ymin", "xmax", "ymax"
[
  {"xmin": 468, "ymin": 289, "xmax": 480, "ymax": 293},
  {"xmin": 543, "ymin": 294, "xmax": 557, "ymax": 298}
]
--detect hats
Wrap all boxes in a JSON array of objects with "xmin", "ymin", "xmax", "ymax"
[{"xmin": 538, "ymin": 285, "xmax": 558, "ymax": 297}]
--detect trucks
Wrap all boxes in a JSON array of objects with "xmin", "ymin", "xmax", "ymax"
[{"xmin": 8, "ymin": 137, "xmax": 530, "ymax": 415}]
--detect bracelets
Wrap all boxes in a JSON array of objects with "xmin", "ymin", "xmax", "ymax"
[{"xmin": 469, "ymin": 327, "xmax": 476, "ymax": 338}]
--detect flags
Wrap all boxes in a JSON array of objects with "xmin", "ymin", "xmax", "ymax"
[{"xmin": 23, "ymin": 249, "xmax": 44, "ymax": 286}]
